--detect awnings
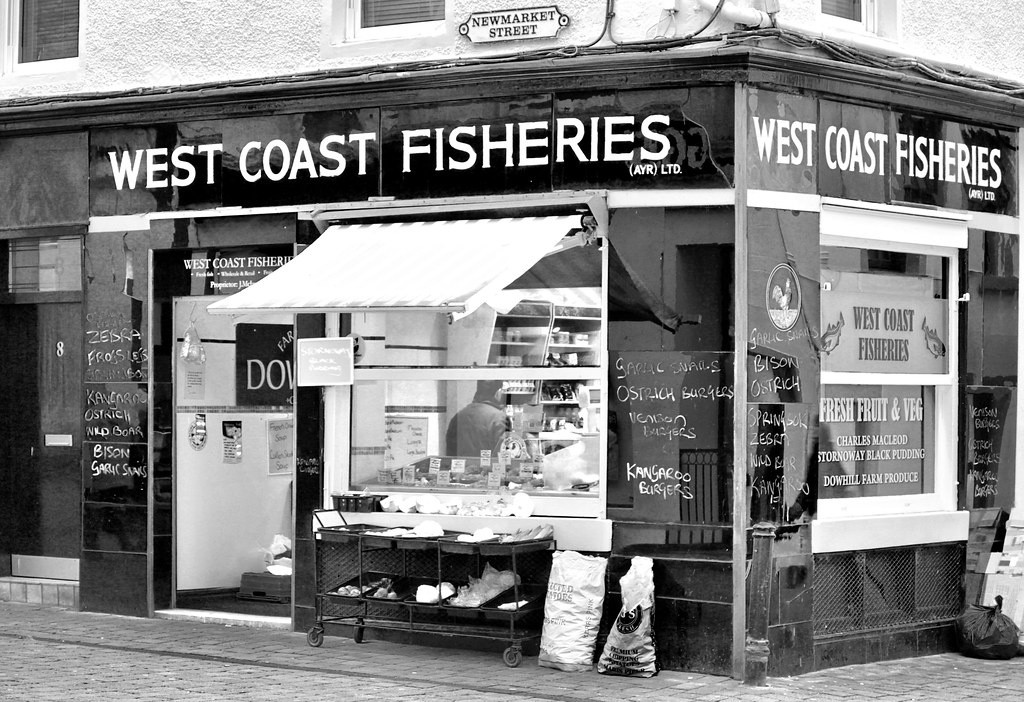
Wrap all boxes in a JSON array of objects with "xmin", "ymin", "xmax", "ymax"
[
  {"xmin": 205, "ymin": 214, "xmax": 575, "ymax": 316},
  {"xmin": 606, "ymin": 239, "xmax": 680, "ymax": 337}
]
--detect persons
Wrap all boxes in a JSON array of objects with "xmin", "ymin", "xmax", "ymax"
[{"xmin": 445, "ymin": 380, "xmax": 512, "ymax": 457}]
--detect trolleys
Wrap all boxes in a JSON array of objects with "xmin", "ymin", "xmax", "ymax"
[{"xmin": 305, "ymin": 509, "xmax": 558, "ymax": 669}]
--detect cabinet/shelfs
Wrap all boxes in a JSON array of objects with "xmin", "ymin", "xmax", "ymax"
[{"xmin": 444, "ymin": 300, "xmax": 602, "ymax": 452}]
